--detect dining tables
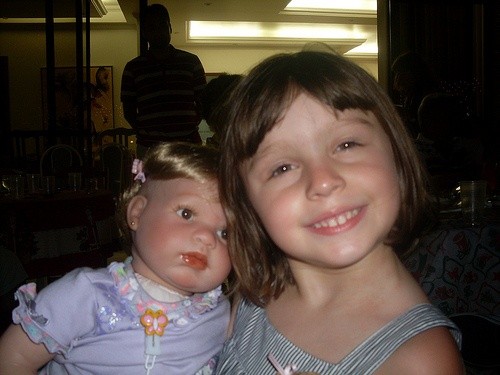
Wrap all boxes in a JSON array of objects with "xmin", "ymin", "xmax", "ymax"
[{"xmin": 0, "ymin": 187, "xmax": 121, "ymax": 311}]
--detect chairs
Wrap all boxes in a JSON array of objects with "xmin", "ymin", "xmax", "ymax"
[{"xmin": 8, "ymin": 128, "xmax": 139, "ymax": 170}]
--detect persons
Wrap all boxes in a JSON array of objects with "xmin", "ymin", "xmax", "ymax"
[
  {"xmin": 119, "ymin": 3, "xmax": 208, "ymax": 171},
  {"xmin": 210, "ymin": 48, "xmax": 469, "ymax": 375},
  {"xmin": 0, "ymin": 139, "xmax": 236, "ymax": 374},
  {"xmin": 392, "ymin": 51, "xmax": 436, "ymax": 138},
  {"xmin": 415, "ymin": 93, "xmax": 477, "ymax": 177}
]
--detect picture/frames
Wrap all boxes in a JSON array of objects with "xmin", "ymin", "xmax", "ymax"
[
  {"xmin": 40, "ymin": 65, "xmax": 115, "ymax": 148},
  {"xmin": 402, "ymin": 222, "xmax": 500, "ymax": 325}
]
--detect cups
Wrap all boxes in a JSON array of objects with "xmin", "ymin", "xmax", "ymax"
[
  {"xmin": 68, "ymin": 173, "xmax": 81, "ymax": 193},
  {"xmin": 15, "ymin": 174, "xmax": 57, "ymax": 199},
  {"xmin": 84, "ymin": 176, "xmax": 99, "ymax": 194},
  {"xmin": 458, "ymin": 181, "xmax": 487, "ymax": 222}
]
